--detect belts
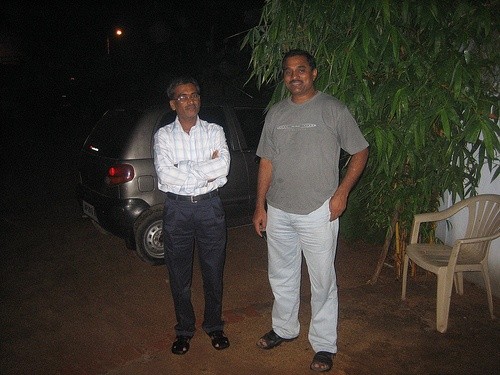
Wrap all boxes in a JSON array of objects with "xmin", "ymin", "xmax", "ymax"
[{"xmin": 167, "ymin": 189, "xmax": 219, "ymax": 203}]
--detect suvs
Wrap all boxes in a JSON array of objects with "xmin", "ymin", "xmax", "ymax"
[{"xmin": 77, "ymin": 106, "xmax": 273, "ymax": 267}]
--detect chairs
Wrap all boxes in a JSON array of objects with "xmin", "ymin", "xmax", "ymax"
[{"xmin": 402, "ymin": 194, "xmax": 500, "ymax": 333}]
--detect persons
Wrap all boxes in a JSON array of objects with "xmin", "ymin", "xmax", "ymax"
[
  {"xmin": 252, "ymin": 49, "xmax": 370, "ymax": 372},
  {"xmin": 154, "ymin": 74, "xmax": 231, "ymax": 355}
]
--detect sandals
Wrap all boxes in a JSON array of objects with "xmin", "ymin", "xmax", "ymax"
[
  {"xmin": 172, "ymin": 333, "xmax": 191, "ymax": 355},
  {"xmin": 202, "ymin": 322, "xmax": 230, "ymax": 350},
  {"xmin": 310, "ymin": 351, "xmax": 337, "ymax": 373},
  {"xmin": 256, "ymin": 329, "xmax": 300, "ymax": 350}
]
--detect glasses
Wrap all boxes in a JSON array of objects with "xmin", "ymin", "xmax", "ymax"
[{"xmin": 169, "ymin": 94, "xmax": 201, "ymax": 102}]
